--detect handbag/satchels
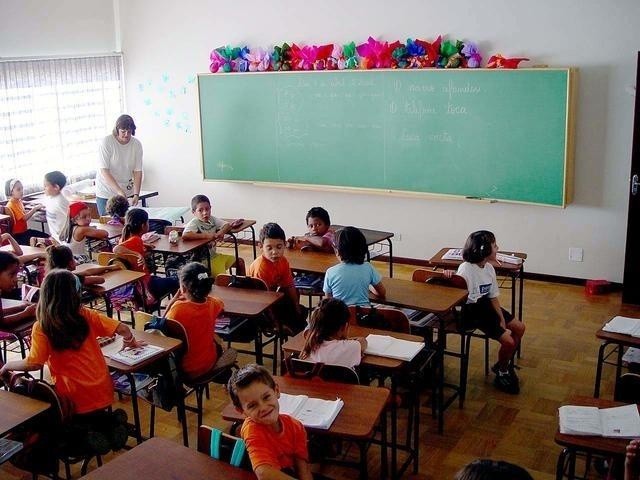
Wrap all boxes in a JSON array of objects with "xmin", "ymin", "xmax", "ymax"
[{"xmin": 105, "ymin": 284, "xmax": 143, "ymax": 312}]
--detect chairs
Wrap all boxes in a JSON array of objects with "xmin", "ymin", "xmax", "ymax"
[{"xmin": 614, "ymin": 373, "xmax": 639, "ymax": 404}]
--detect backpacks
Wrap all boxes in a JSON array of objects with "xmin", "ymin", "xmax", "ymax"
[
  {"xmin": 282, "ymin": 355, "xmax": 325, "ymax": 382},
  {"xmin": 111, "ymin": 315, "xmax": 183, "ymax": 412},
  {"xmin": 164, "ymin": 254, "xmax": 186, "ymax": 288},
  {"xmin": 6, "ymin": 373, "xmax": 60, "ymax": 479}
]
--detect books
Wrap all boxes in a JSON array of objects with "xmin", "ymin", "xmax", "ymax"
[
  {"xmin": 276, "ymin": 393, "xmax": 345, "ymax": 430},
  {"xmin": 557, "ymin": 403, "xmax": 640, "ymax": 439},
  {"xmin": 602, "ymin": 315, "xmax": 640, "ymax": 339},
  {"xmin": 441, "ymin": 248, "xmax": 464, "ymax": 261},
  {"xmin": 363, "ymin": 333, "xmax": 426, "ymax": 363}
]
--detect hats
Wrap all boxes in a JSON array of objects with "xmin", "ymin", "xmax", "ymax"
[{"xmin": 69, "ymin": 202, "xmax": 88, "ymax": 219}]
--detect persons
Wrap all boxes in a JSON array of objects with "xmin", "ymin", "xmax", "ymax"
[
  {"xmin": 442, "ymin": 229, "xmax": 526, "ymax": 395},
  {"xmin": 299, "ymin": 297, "xmax": 368, "ymax": 372},
  {"xmin": 0, "ymin": 171, "xmax": 237, "ymax": 461},
  {"xmin": 453, "ymin": 458, "xmax": 535, "ymax": 480},
  {"xmin": 246, "ymin": 221, "xmax": 309, "ymax": 337},
  {"xmin": 321, "ymin": 225, "xmax": 386, "ymax": 309},
  {"xmin": 227, "ymin": 362, "xmax": 332, "ymax": 480},
  {"xmin": 94, "ymin": 113, "xmax": 144, "ymax": 216},
  {"xmin": 624, "ymin": 439, "xmax": 640, "ymax": 480},
  {"xmin": 286, "ymin": 206, "xmax": 337, "ymax": 254}
]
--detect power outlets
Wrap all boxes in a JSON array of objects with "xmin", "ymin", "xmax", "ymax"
[{"xmin": 569, "ymin": 248, "xmax": 582, "ymax": 263}]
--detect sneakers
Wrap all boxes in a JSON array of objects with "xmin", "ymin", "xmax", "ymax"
[{"xmin": 491, "ymin": 362, "xmax": 520, "ymax": 395}]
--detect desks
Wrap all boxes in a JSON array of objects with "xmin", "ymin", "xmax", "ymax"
[
  {"xmin": 591, "ymin": 312, "xmax": 640, "ymax": 399},
  {"xmin": 555, "ymin": 396, "xmax": 639, "ymax": 480}
]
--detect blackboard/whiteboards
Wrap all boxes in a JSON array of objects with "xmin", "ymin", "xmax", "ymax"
[{"xmin": 197, "ymin": 67, "xmax": 570, "ymax": 210}]
